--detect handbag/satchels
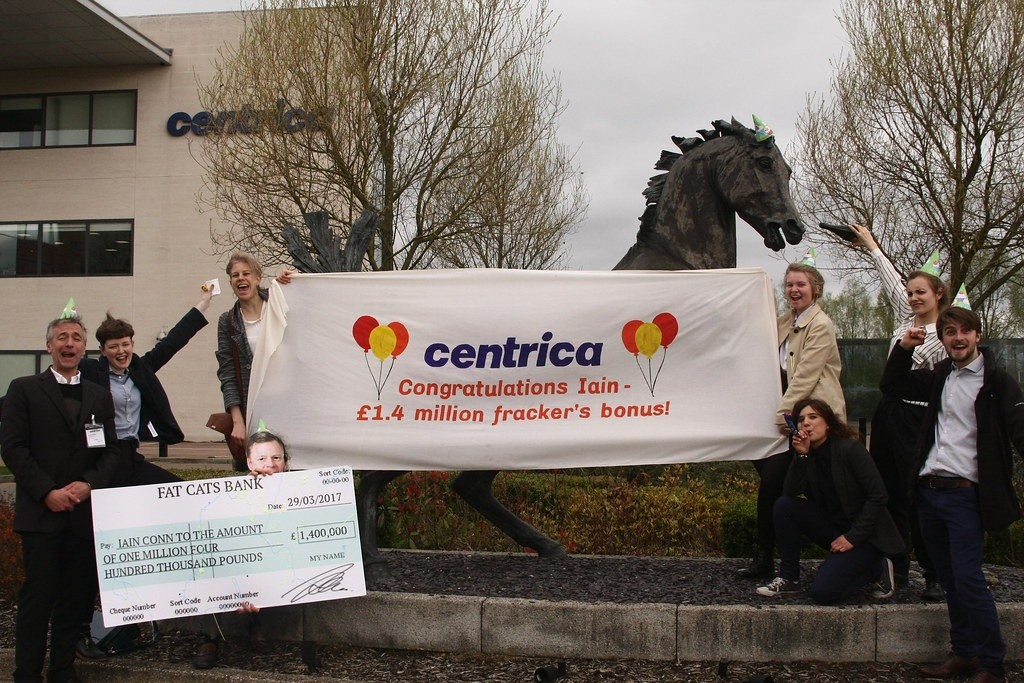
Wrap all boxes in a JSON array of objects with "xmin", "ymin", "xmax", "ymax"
[
  {"xmin": 206, "ymin": 410, "xmax": 249, "ymax": 463},
  {"xmin": 90, "ymin": 610, "xmax": 144, "ymax": 654}
]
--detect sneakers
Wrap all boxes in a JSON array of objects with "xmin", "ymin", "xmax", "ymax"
[
  {"xmin": 756, "ymin": 576, "xmax": 805, "ymax": 596},
  {"xmin": 872, "ymin": 558, "xmax": 895, "ymax": 598}
]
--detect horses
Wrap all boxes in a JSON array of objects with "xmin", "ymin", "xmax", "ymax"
[{"xmin": 279, "ymin": 113, "xmax": 805, "ymax": 566}]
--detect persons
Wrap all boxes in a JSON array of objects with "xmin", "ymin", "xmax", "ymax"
[
  {"xmin": 215, "ymin": 251, "xmax": 297, "ymax": 468},
  {"xmin": 1, "ymin": 315, "xmax": 122, "ymax": 683},
  {"xmin": 76, "ymin": 284, "xmax": 215, "ymax": 659},
  {"xmin": 193, "ymin": 419, "xmax": 289, "ymax": 669},
  {"xmin": 848, "ymin": 223, "xmax": 950, "ymax": 603},
  {"xmin": 756, "ymin": 400, "xmax": 904, "ymax": 605},
  {"xmin": 880, "ymin": 281, "xmax": 1024, "ymax": 683},
  {"xmin": 736, "ymin": 264, "xmax": 848, "ymax": 580}
]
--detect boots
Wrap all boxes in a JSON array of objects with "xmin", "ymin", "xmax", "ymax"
[{"xmin": 738, "ymin": 543, "xmax": 774, "ymax": 579}]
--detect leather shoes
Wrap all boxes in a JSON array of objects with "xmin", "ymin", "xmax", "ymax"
[
  {"xmin": 968, "ymin": 670, "xmax": 1006, "ymax": 683},
  {"xmin": 920, "ymin": 654, "xmax": 979, "ymax": 677}
]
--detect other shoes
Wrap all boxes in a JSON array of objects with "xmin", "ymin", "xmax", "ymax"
[
  {"xmin": 194, "ymin": 642, "xmax": 216, "ymax": 669},
  {"xmin": 921, "ymin": 574, "xmax": 945, "ymax": 601}
]
